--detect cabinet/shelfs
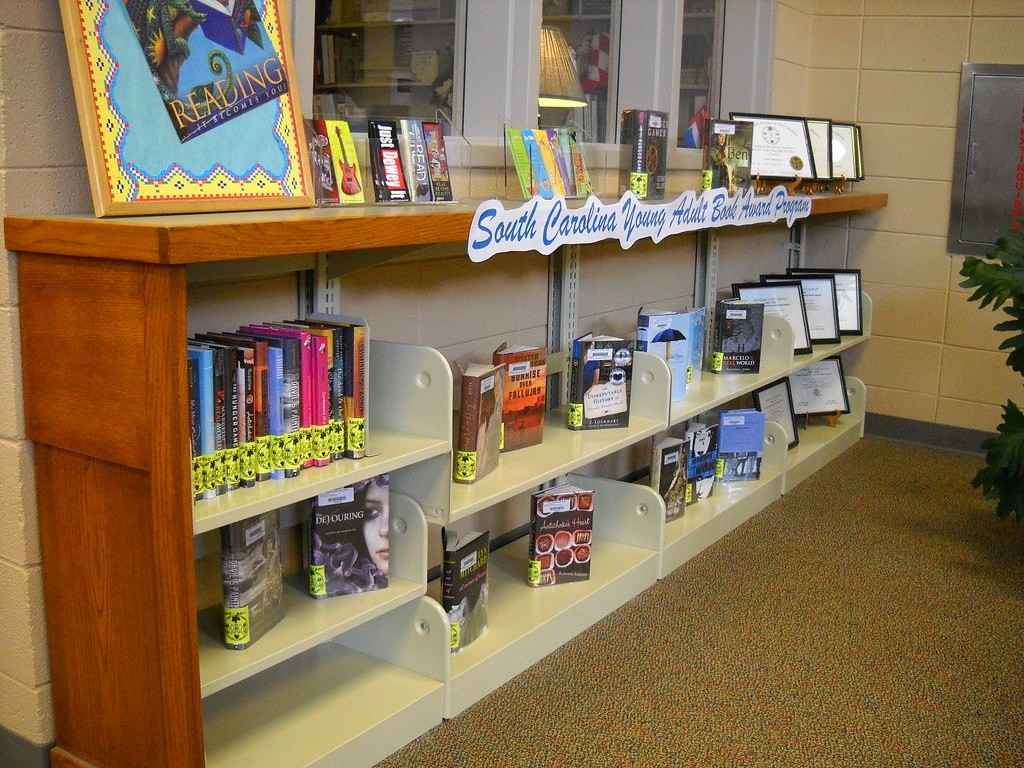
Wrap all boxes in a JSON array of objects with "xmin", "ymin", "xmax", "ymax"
[
  {"xmin": 312, "ymin": 0, "xmax": 714, "ymax": 122},
  {"xmin": 4, "ymin": 193, "xmax": 890, "ymax": 768}
]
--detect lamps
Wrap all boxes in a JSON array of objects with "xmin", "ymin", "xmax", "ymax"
[{"xmin": 537, "ymin": 25, "xmax": 589, "ymax": 129}]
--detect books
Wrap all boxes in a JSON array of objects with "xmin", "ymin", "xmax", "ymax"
[
  {"xmin": 649, "ymin": 435, "xmax": 691, "ymax": 524},
  {"xmin": 575, "ymin": 93, "xmax": 606, "ymax": 143},
  {"xmin": 527, "ymin": 482, "xmax": 595, "ymax": 588},
  {"xmin": 492, "ymin": 342, "xmax": 548, "ymax": 452},
  {"xmin": 636, "ymin": 305, "xmax": 706, "ymax": 403},
  {"xmin": 440, "ymin": 527, "xmax": 490, "ymax": 653},
  {"xmin": 222, "ymin": 509, "xmax": 293, "ymax": 643},
  {"xmin": 618, "ymin": 110, "xmax": 671, "ymax": 198},
  {"xmin": 568, "ymin": 332, "xmax": 634, "ymax": 431},
  {"xmin": 453, "ymin": 361, "xmax": 505, "ymax": 485},
  {"xmin": 684, "ymin": 421, "xmax": 719, "ymax": 507},
  {"xmin": 715, "ymin": 408, "xmax": 764, "ymax": 483},
  {"xmin": 186, "ymin": 320, "xmax": 365, "ymax": 506},
  {"xmin": 710, "ymin": 298, "xmax": 764, "ymax": 375},
  {"xmin": 309, "ymin": 473, "xmax": 390, "ymax": 599},
  {"xmin": 700, "ymin": 118, "xmax": 753, "ymax": 198},
  {"xmin": 313, "ymin": 34, "xmax": 369, "ymax": 130}
]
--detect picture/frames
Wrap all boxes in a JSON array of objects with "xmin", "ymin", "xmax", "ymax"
[{"xmin": 60, "ymin": 0, "xmax": 316, "ymax": 218}]
